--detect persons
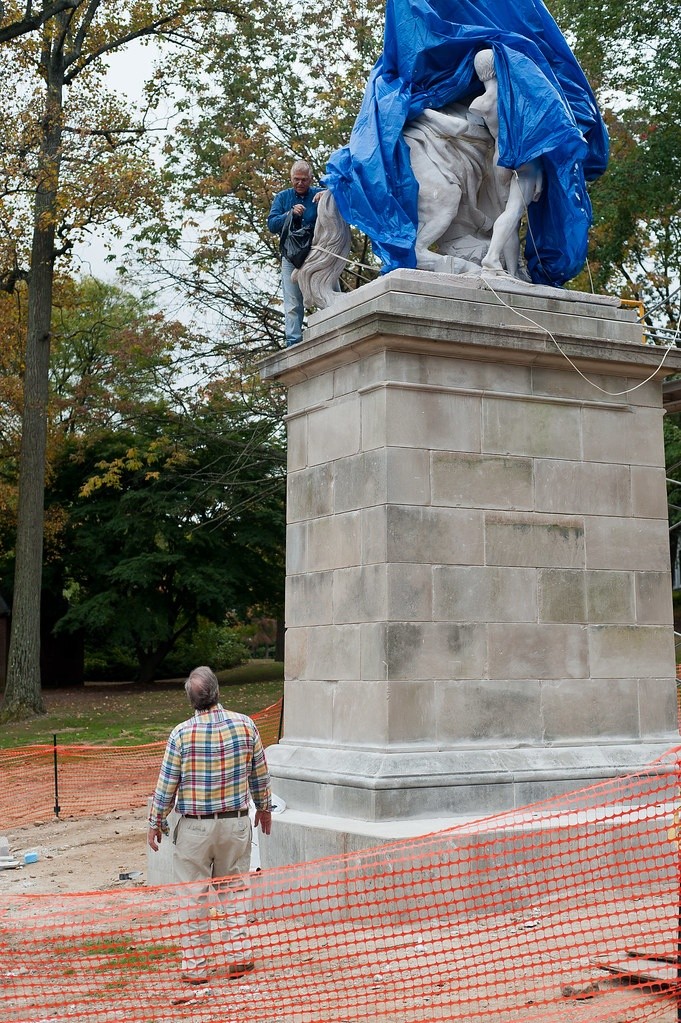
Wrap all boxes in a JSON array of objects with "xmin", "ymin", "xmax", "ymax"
[
  {"xmin": 268, "ymin": 161, "xmax": 341, "ymax": 347},
  {"xmin": 469, "ymin": 49, "xmax": 542, "ymax": 275},
  {"xmin": 147, "ymin": 665, "xmax": 272, "ymax": 984}
]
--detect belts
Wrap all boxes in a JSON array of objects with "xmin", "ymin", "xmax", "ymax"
[{"xmin": 184, "ymin": 810, "xmax": 248, "ymax": 819}]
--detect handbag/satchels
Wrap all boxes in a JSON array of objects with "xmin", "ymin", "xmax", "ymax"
[{"xmin": 279, "ymin": 206, "xmax": 314, "ymax": 269}]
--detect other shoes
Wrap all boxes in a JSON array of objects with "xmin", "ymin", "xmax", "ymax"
[
  {"xmin": 228, "ymin": 958, "xmax": 253, "ymax": 980},
  {"xmin": 180, "ymin": 973, "xmax": 208, "ymax": 984}
]
